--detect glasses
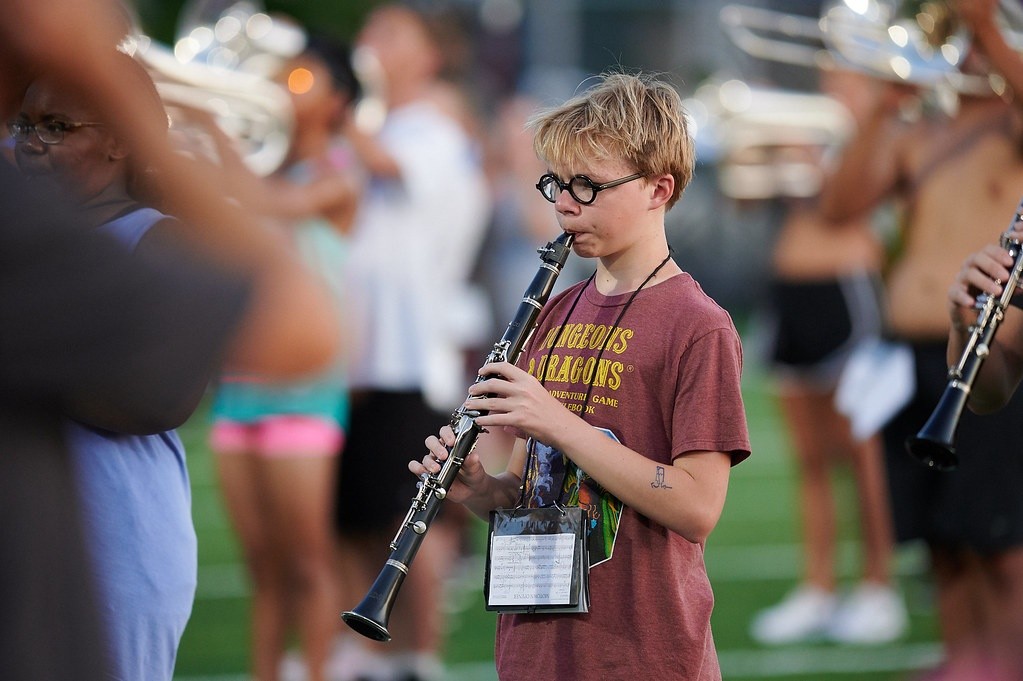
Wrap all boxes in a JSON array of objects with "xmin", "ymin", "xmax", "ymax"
[
  {"xmin": 536, "ymin": 169, "xmax": 653, "ymax": 203},
  {"xmin": 7, "ymin": 120, "xmax": 104, "ymax": 146}
]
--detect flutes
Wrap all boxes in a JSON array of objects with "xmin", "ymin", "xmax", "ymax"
[
  {"xmin": 906, "ymin": 207, "xmax": 1023, "ymax": 468},
  {"xmin": 342, "ymin": 231, "xmax": 577, "ymax": 641}
]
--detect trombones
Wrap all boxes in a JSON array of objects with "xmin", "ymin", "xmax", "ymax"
[{"xmin": 709, "ymin": 1, "xmax": 1013, "ymax": 118}]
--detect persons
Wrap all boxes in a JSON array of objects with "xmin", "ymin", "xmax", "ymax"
[
  {"xmin": 408, "ymin": 76, "xmax": 752, "ymax": 681},
  {"xmin": 0, "ymin": 2, "xmax": 488, "ymax": 681}
]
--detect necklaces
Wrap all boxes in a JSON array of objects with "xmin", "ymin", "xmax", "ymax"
[{"xmin": 749, "ymin": 0, "xmax": 1023, "ymax": 681}]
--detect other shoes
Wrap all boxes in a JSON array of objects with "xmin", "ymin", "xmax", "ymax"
[
  {"xmin": 754, "ymin": 590, "xmax": 831, "ymax": 645},
  {"xmin": 827, "ymin": 587, "xmax": 909, "ymax": 648}
]
